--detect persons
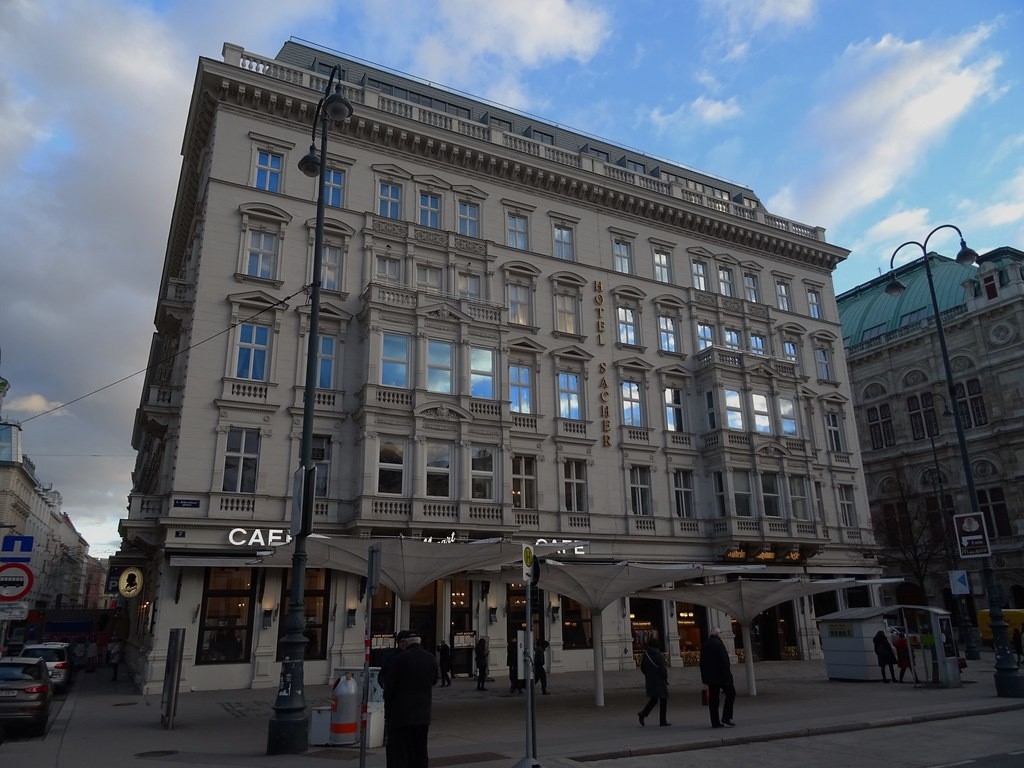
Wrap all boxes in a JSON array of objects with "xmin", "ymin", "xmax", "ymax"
[
  {"xmin": 377, "ymin": 630, "xmax": 411, "ymax": 748},
  {"xmin": 637, "ymin": 638, "xmax": 673, "ymax": 728},
  {"xmin": 893, "ymin": 631, "xmax": 921, "ymax": 684},
  {"xmin": 109, "ymin": 637, "xmax": 123, "ymax": 681},
  {"xmin": 699, "ymin": 626, "xmax": 737, "ymax": 729},
  {"xmin": 534, "ymin": 641, "xmax": 551, "ymax": 696},
  {"xmin": 872, "ymin": 630, "xmax": 899, "ymax": 684},
  {"xmin": 205, "ymin": 618, "xmax": 245, "ymax": 664},
  {"xmin": 943, "ymin": 632, "xmax": 963, "ymax": 674},
  {"xmin": 505, "ymin": 634, "xmax": 524, "ymax": 697},
  {"xmin": 1011, "ymin": 628, "xmax": 1024, "ymax": 665},
  {"xmin": 383, "ymin": 630, "xmax": 439, "ymax": 768},
  {"xmin": 475, "ymin": 638, "xmax": 490, "ymax": 691},
  {"xmin": 73, "ymin": 637, "xmax": 87, "ymax": 670},
  {"xmin": 436, "ymin": 640, "xmax": 452, "ymax": 688},
  {"xmin": 105, "ymin": 637, "xmax": 115, "ymax": 665},
  {"xmin": 83, "ymin": 632, "xmax": 99, "ymax": 674}
]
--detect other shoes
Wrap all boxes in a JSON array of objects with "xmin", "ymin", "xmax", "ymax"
[
  {"xmin": 476, "ymin": 686, "xmax": 488, "ymax": 691},
  {"xmin": 542, "ymin": 691, "xmax": 551, "ymax": 695},
  {"xmin": 439, "ymin": 681, "xmax": 452, "ymax": 688}
]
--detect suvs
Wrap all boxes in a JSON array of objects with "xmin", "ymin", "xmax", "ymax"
[
  {"xmin": 0, "ymin": 656, "xmax": 52, "ymax": 737},
  {"xmin": 19, "ymin": 644, "xmax": 67, "ymax": 684}
]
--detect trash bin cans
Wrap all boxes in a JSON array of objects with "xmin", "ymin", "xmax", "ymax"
[{"xmin": 329, "ymin": 675, "xmax": 360, "ymax": 745}]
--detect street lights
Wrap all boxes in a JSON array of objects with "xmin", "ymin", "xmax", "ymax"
[
  {"xmin": 266, "ymin": 61, "xmax": 353, "ymax": 754},
  {"xmin": 883, "ymin": 223, "xmax": 1024, "ymax": 698}
]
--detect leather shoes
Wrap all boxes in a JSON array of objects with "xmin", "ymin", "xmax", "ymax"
[
  {"xmin": 638, "ymin": 711, "xmax": 645, "ymax": 726},
  {"xmin": 721, "ymin": 718, "xmax": 736, "ymax": 726},
  {"xmin": 660, "ymin": 722, "xmax": 671, "ymax": 726},
  {"xmin": 715, "ymin": 723, "xmax": 724, "ymax": 729}
]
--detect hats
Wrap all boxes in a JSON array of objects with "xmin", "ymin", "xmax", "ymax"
[{"xmin": 396, "ymin": 630, "xmax": 421, "ymax": 643}]
